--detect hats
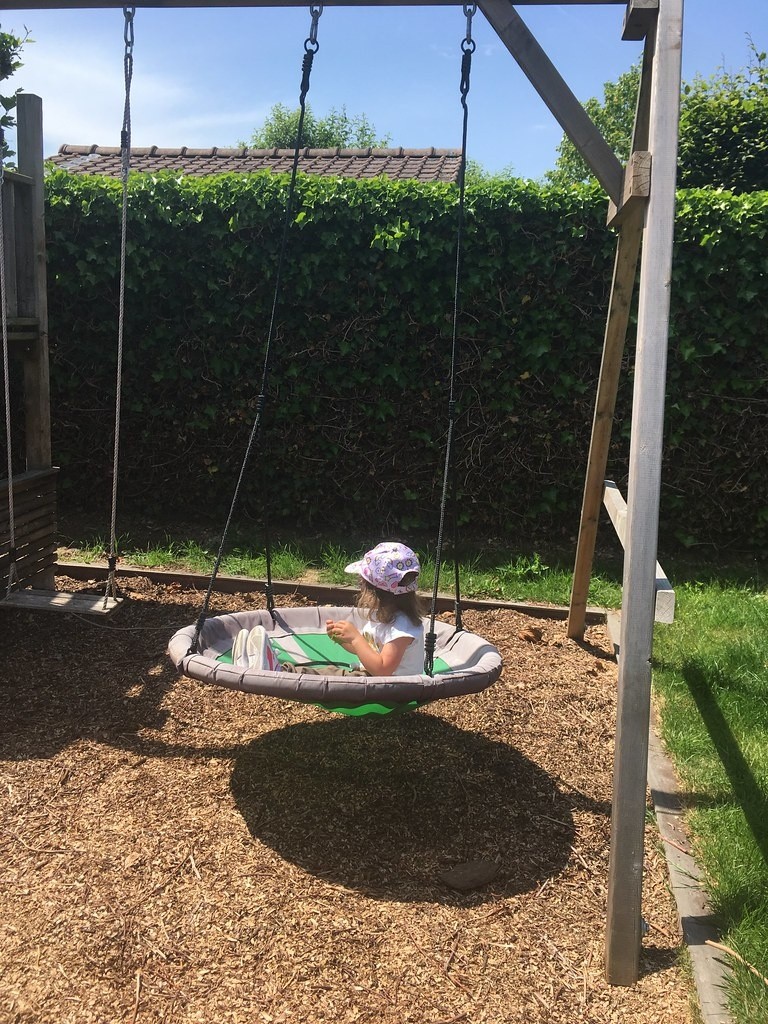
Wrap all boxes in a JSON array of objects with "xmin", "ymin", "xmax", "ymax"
[{"xmin": 344, "ymin": 542, "xmax": 420, "ymax": 595}]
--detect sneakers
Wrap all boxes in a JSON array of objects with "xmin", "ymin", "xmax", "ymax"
[
  {"xmin": 232, "ymin": 627, "xmax": 250, "ymax": 669},
  {"xmin": 247, "ymin": 625, "xmax": 280, "ymax": 671}
]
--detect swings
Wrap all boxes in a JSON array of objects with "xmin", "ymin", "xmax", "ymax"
[{"xmin": 162, "ymin": 3, "xmax": 503, "ymax": 707}]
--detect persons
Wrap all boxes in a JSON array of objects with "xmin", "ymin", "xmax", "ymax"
[{"xmin": 230, "ymin": 542, "xmax": 425, "ymax": 677}]
